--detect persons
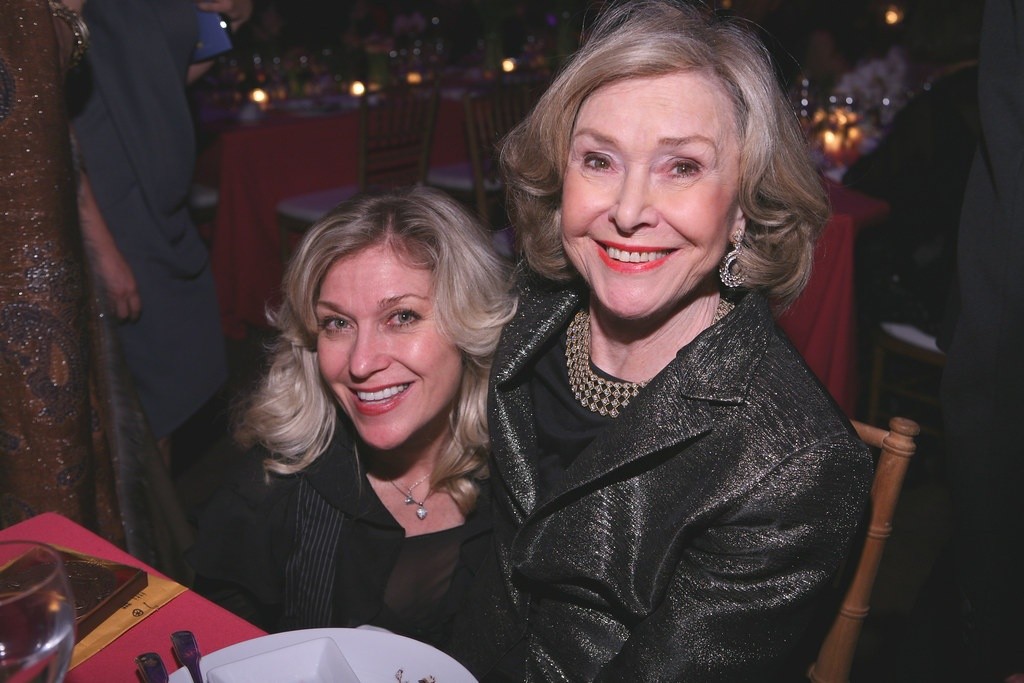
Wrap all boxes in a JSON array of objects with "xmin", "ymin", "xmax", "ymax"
[
  {"xmin": 186, "ymin": 182, "xmax": 520, "ymax": 656},
  {"xmin": 718, "ymin": 1, "xmax": 1024, "ymax": 553},
  {"xmin": 448, "ymin": 0, "xmax": 875, "ymax": 683},
  {"xmin": 0, "ymin": 0, "xmax": 534, "ymax": 564}
]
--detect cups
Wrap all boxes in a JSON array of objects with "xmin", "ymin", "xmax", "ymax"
[{"xmin": 0, "ymin": 540, "xmax": 79, "ymax": 683}]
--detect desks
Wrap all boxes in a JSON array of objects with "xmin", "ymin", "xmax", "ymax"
[
  {"xmin": 195, "ymin": 83, "xmax": 532, "ymax": 342},
  {"xmin": 0, "ymin": 512, "xmax": 270, "ymax": 683},
  {"xmin": 769, "ymin": 177, "xmax": 895, "ymax": 420}
]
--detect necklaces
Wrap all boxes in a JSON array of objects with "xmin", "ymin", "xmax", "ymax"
[
  {"xmin": 558, "ymin": 306, "xmax": 654, "ymax": 420},
  {"xmin": 375, "ymin": 448, "xmax": 439, "ymax": 525}
]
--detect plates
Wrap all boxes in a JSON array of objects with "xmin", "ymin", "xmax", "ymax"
[
  {"xmin": 165, "ymin": 627, "xmax": 480, "ymax": 683},
  {"xmin": 205, "ymin": 636, "xmax": 362, "ymax": 683}
]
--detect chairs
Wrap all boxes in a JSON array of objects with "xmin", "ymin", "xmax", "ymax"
[
  {"xmin": 867, "ymin": 321, "xmax": 948, "ymax": 486},
  {"xmin": 462, "ymin": 78, "xmax": 537, "ymax": 264},
  {"xmin": 273, "ymin": 72, "xmax": 443, "ymax": 270}
]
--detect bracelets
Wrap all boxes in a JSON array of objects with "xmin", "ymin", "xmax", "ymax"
[{"xmin": 48, "ymin": 9, "xmax": 91, "ymax": 64}]
